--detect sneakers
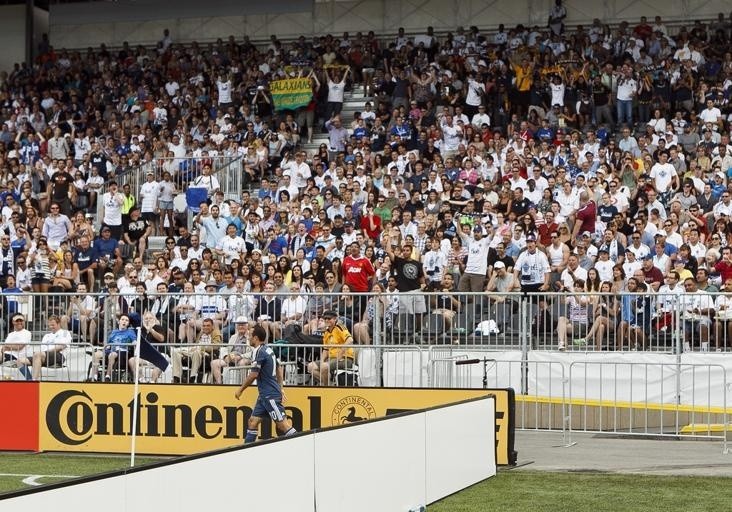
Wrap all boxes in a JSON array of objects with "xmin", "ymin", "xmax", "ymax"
[
  {"xmin": 573, "ymin": 338, "xmax": 587, "ymax": 346},
  {"xmin": 559, "ymin": 340, "xmax": 566, "ymax": 352}
]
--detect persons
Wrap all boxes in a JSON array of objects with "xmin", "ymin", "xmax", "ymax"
[
  {"xmin": 1, "ymin": 0, "xmax": 731, "ymax": 389},
  {"xmin": 234, "ymin": 324, "xmax": 300, "ymax": 443}
]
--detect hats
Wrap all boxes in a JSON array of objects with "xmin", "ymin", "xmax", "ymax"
[
  {"xmin": 494, "ymin": 261, "xmax": 506, "ymax": 270},
  {"xmin": 104, "ymin": 272, "xmax": 115, "ymax": 278},
  {"xmin": 102, "ymin": 227, "xmax": 111, "ymax": 232},
  {"xmin": 13, "ymin": 315, "xmax": 24, "ymax": 321},
  {"xmin": 252, "ymin": 249, "xmax": 262, "ymax": 256},
  {"xmin": 576, "ymin": 230, "xmax": 609, "ymax": 254},
  {"xmin": 322, "ymin": 311, "xmax": 337, "ymax": 319},
  {"xmin": 625, "ymin": 246, "xmax": 683, "ymax": 264},
  {"xmin": 205, "ymin": 279, "xmax": 219, "ymax": 288},
  {"xmin": 85, "ymin": 213, "xmax": 94, "ymax": 219},
  {"xmin": 232, "ymin": 316, "xmax": 249, "ymax": 324},
  {"xmin": 473, "ymin": 225, "xmax": 483, "ymax": 231}
]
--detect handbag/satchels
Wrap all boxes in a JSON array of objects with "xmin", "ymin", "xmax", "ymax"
[
  {"xmin": 719, "ymin": 304, "xmax": 732, "ymax": 318},
  {"xmin": 680, "ymin": 307, "xmax": 702, "ymax": 322}
]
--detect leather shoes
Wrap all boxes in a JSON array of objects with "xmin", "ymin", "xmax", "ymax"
[
  {"xmin": 104, "ymin": 377, "xmax": 111, "ymax": 382},
  {"xmin": 85, "ymin": 378, "xmax": 98, "ymax": 383}
]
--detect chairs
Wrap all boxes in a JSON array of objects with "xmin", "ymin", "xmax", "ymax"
[{"xmin": 389, "ymin": 303, "xmax": 570, "ymax": 344}]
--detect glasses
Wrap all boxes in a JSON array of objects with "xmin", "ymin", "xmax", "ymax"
[
  {"xmin": 106, "ymin": 277, "xmax": 113, "ymax": 280},
  {"xmin": 486, "ymin": 217, "xmax": 559, "ymax": 243},
  {"xmin": 292, "ymin": 146, "xmax": 448, "ymax": 232},
  {"xmin": 501, "ymin": 155, "xmax": 617, "ymax": 196},
  {"xmin": 130, "ymin": 275, "xmax": 137, "ymax": 279},
  {"xmin": 633, "ymin": 185, "xmax": 721, "ymax": 241},
  {"xmin": 13, "ymin": 319, "xmax": 24, "ymax": 323},
  {"xmin": 167, "ymin": 240, "xmax": 197, "ymax": 281},
  {"xmin": 252, "ymin": 252, "xmax": 258, "ymax": 256},
  {"xmin": 2, "ymin": 197, "xmax": 59, "ymax": 265}
]
